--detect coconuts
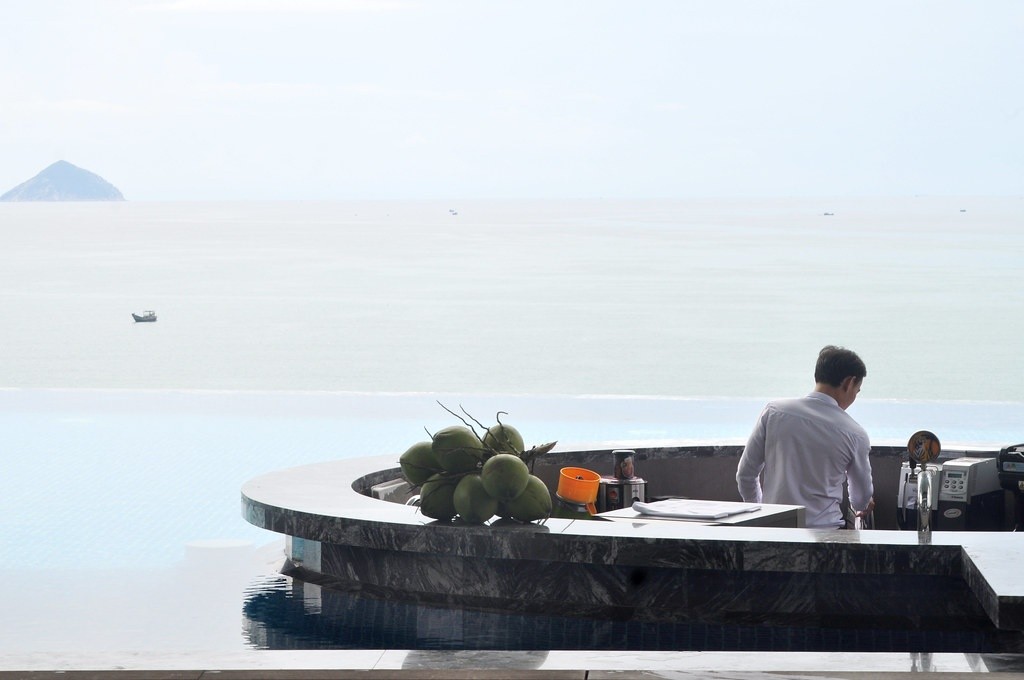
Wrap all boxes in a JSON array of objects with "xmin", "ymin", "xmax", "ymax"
[{"xmin": 400, "ymin": 425, "xmax": 552, "ymax": 521}]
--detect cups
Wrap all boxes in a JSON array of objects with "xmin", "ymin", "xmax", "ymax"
[{"xmin": 612, "ymin": 450, "xmax": 636, "ymax": 480}]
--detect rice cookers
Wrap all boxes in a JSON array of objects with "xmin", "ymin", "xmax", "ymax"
[{"xmin": 595, "ymin": 476, "xmax": 647, "ymax": 513}]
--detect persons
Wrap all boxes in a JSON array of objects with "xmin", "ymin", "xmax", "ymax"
[{"xmin": 736, "ymin": 345, "xmax": 875, "ymax": 529}]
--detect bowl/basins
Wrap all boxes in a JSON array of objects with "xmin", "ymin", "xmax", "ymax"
[{"xmin": 558, "ymin": 467, "xmax": 601, "ymax": 504}]
